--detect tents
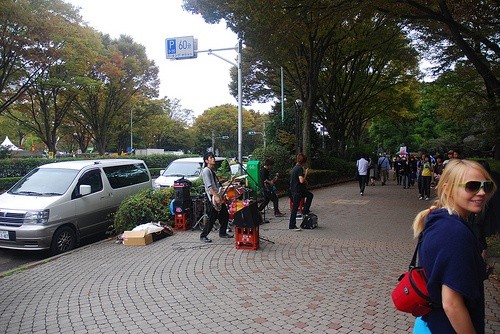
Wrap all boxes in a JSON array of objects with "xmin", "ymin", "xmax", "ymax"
[{"xmin": 0, "ymin": 135, "xmax": 23, "ymax": 159}]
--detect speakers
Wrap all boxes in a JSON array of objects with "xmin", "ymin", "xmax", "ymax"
[
  {"xmin": 300, "ymin": 212, "xmax": 318, "ymax": 230},
  {"xmin": 233, "ymin": 204, "xmax": 263, "ymax": 228}
]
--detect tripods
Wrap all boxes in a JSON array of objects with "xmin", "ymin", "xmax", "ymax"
[{"xmin": 192, "ymin": 193, "xmax": 218, "ymax": 231}]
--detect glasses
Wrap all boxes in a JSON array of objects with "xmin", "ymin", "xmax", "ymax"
[{"xmin": 453, "ymin": 181, "xmax": 494, "ymax": 194}]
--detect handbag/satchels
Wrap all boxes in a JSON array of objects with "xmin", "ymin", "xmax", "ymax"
[
  {"xmin": 378, "ymin": 164, "xmax": 381, "ymax": 169},
  {"xmin": 391, "ymin": 267, "xmax": 430, "ymax": 317}
]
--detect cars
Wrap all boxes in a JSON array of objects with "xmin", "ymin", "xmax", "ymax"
[{"xmin": 153, "ymin": 154, "xmax": 252, "ymax": 191}]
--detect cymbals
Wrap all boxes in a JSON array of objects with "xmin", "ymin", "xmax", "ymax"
[{"xmin": 235, "ymin": 175, "xmax": 249, "ymax": 179}]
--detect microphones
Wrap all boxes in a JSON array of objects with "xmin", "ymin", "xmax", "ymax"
[{"xmin": 236, "ymin": 161, "xmax": 244, "ymax": 166}]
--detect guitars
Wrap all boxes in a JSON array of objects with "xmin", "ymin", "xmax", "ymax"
[
  {"xmin": 265, "ymin": 172, "xmax": 279, "ymax": 193},
  {"xmin": 211, "ymin": 176, "xmax": 235, "ymax": 212}
]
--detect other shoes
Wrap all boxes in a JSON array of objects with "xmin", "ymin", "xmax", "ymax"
[
  {"xmin": 424, "ymin": 195, "xmax": 428, "ymax": 199},
  {"xmin": 274, "ymin": 209, "xmax": 283, "ymax": 216},
  {"xmin": 425, "ymin": 198, "xmax": 430, "ymax": 201},
  {"xmin": 289, "ymin": 226, "xmax": 300, "ymax": 230},
  {"xmin": 200, "ymin": 237, "xmax": 212, "ymax": 242},
  {"xmin": 360, "ymin": 192, "xmax": 363, "ymax": 196},
  {"xmin": 219, "ymin": 234, "xmax": 231, "ymax": 238},
  {"xmin": 418, "ymin": 194, "xmax": 421, "ymax": 197},
  {"xmin": 382, "ymin": 182, "xmax": 384, "ymax": 186},
  {"xmin": 419, "ymin": 197, "xmax": 423, "ymax": 199}
]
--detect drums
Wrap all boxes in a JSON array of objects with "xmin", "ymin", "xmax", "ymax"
[
  {"xmin": 222, "ymin": 186, "xmax": 257, "ymax": 219},
  {"xmin": 218, "ymin": 175, "xmax": 227, "ymax": 183}
]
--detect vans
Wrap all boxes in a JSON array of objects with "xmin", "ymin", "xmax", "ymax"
[{"xmin": 1, "ymin": 159, "xmax": 152, "ymax": 254}]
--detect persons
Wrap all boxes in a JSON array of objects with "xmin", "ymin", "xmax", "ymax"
[
  {"xmin": 288, "ymin": 152, "xmax": 314, "ymax": 231},
  {"xmin": 257, "ymin": 158, "xmax": 286, "ymax": 216},
  {"xmin": 355, "ymin": 148, "xmax": 496, "ymax": 264},
  {"xmin": 412, "ymin": 157, "xmax": 497, "ymax": 334},
  {"xmin": 200, "ymin": 152, "xmax": 234, "ymax": 243}
]
